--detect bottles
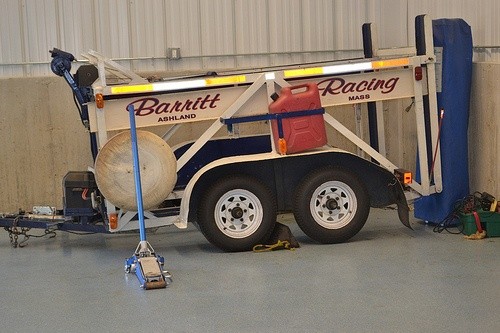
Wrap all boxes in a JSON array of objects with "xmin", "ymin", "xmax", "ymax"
[{"xmin": 269, "ymin": 82, "xmax": 327, "ymax": 154}]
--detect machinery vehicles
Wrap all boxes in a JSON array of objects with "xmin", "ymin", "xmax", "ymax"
[{"xmin": 1, "ymin": 13, "xmax": 444, "ymax": 252}]
